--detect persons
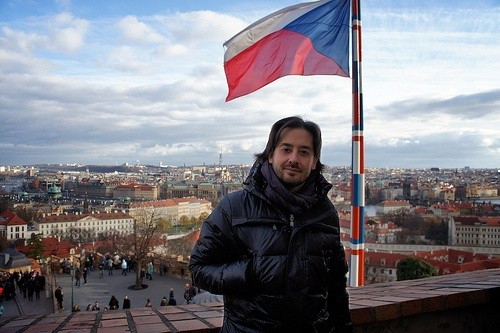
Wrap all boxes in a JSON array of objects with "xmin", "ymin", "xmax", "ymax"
[
  {"xmin": 99, "ymin": 261, "xmax": 105, "ymax": 277},
  {"xmin": 123, "ymin": 295, "xmax": 130, "ymax": 309},
  {"xmin": 13, "ymin": 270, "xmax": 45, "ymax": 301},
  {"xmin": 122, "ymin": 260, "xmax": 127, "ymax": 276},
  {"xmin": 107, "ymin": 258, "xmax": 113, "ymax": 276},
  {"xmin": 55, "ymin": 287, "xmax": 64, "ymax": 310},
  {"xmin": 75, "ymin": 268, "xmax": 82, "ymax": 287},
  {"xmin": 83, "ymin": 268, "xmax": 87, "ymax": 283},
  {"xmin": 184, "ymin": 283, "xmax": 196, "ymax": 304},
  {"xmin": 106, "ymin": 254, "xmax": 130, "ymax": 272},
  {"xmin": 62, "ymin": 257, "xmax": 94, "ymax": 275},
  {"xmin": 140, "ymin": 262, "xmax": 153, "ymax": 283},
  {"xmin": 72, "ymin": 295, "xmax": 119, "ymax": 313},
  {"xmin": 160, "ymin": 288, "xmax": 176, "ymax": 306},
  {"xmin": 187, "ymin": 117, "xmax": 350, "ymax": 333},
  {"xmin": 145, "ymin": 299, "xmax": 152, "ymax": 307},
  {"xmin": 0, "ymin": 272, "xmax": 16, "ymax": 317}
]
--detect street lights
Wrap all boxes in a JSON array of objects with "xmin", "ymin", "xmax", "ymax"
[{"xmin": 69, "ymin": 265, "xmax": 76, "ymax": 314}]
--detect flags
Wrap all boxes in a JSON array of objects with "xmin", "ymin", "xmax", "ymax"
[{"xmin": 223, "ymin": 0, "xmax": 351, "ymax": 102}]
